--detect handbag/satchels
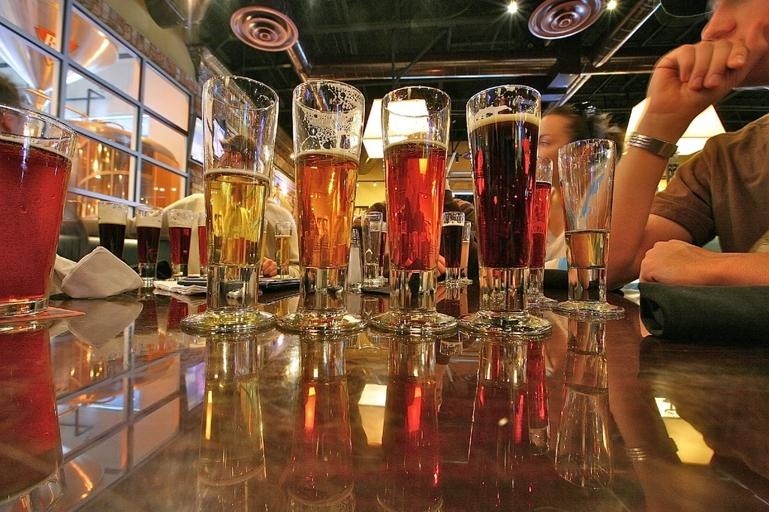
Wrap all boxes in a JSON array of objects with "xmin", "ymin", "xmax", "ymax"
[
  {"xmin": 623, "ymin": 437, "xmax": 678, "ymax": 462},
  {"xmin": 628, "ymin": 132, "xmax": 679, "ymax": 159}
]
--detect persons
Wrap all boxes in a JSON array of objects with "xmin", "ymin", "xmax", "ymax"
[
  {"xmin": 569, "ymin": 0, "xmax": 769, "ymax": 294},
  {"xmin": 594, "ymin": 294, "xmax": 767, "ymax": 510},
  {"xmin": 352, "ymin": 181, "xmax": 480, "ymax": 287},
  {"xmin": 1, "ymin": 72, "xmax": 25, "ymax": 136},
  {"xmin": 164, "ymin": 136, "xmax": 299, "ymax": 277},
  {"xmin": 436, "ymin": 287, "xmax": 448, "ymax": 304},
  {"xmin": 537, "ymin": 310, "xmax": 593, "ymax": 387},
  {"xmin": 532, "ymin": 100, "xmax": 625, "ymax": 268}
]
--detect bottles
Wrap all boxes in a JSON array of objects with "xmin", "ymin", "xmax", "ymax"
[{"xmin": 219, "ymin": 134, "xmax": 258, "ymax": 159}]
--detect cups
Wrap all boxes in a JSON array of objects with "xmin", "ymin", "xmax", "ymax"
[
  {"xmin": 196, "ymin": 76, "xmax": 280, "ymax": 340},
  {"xmin": 355, "ymin": 209, "xmax": 385, "ymax": 293},
  {"xmin": 370, "ymin": 83, "xmax": 459, "ymax": 333},
  {"xmin": 463, "ymin": 82, "xmax": 554, "ymax": 339},
  {"xmin": 572, "ymin": 100, "xmax": 597, "ymax": 138},
  {"xmin": 440, "ymin": 209, "xmax": 470, "ymax": 293},
  {"xmin": 165, "ymin": 207, "xmax": 191, "ymax": 285},
  {"xmin": 555, "ymin": 139, "xmax": 624, "ymax": 324},
  {"xmin": 284, "ymin": 76, "xmax": 370, "ymax": 335},
  {"xmin": 130, "ymin": 205, "xmax": 159, "ymax": 291},
  {"xmin": 98, "ymin": 205, "xmax": 123, "ymax": 261}
]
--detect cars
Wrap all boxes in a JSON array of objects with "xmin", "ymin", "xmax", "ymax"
[{"xmin": 0, "ymin": 104, "xmax": 76, "ymax": 318}]
